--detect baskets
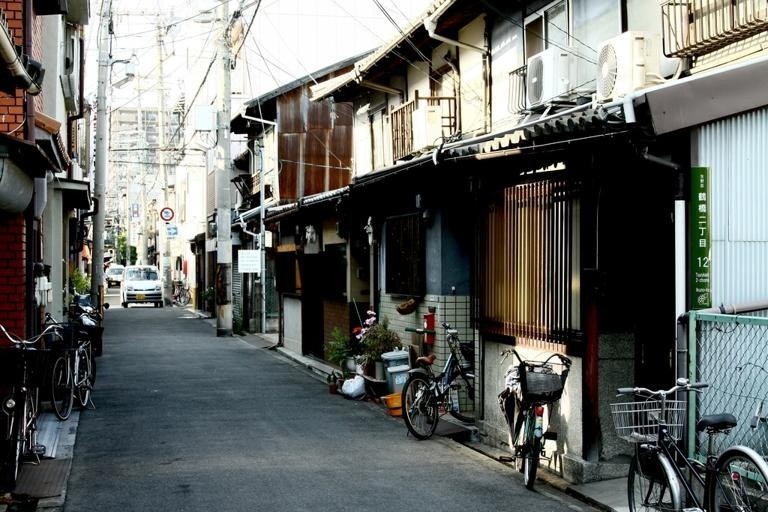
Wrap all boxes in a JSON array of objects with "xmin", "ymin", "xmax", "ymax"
[
  {"xmin": 4, "ymin": 346, "xmax": 50, "ymax": 387},
  {"xmin": 460, "ymin": 340, "xmax": 474, "ymax": 363},
  {"xmin": 519, "ymin": 360, "xmax": 569, "ymax": 401},
  {"xmin": 43, "ymin": 322, "xmax": 104, "ymax": 357},
  {"xmin": 611, "ymin": 400, "xmax": 688, "ymax": 444}
]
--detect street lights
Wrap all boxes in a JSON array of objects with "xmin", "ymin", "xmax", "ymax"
[
  {"xmin": 192, "ymin": 0, "xmax": 237, "ymax": 338},
  {"xmin": 91, "ymin": 1, "xmax": 137, "ymax": 329}
]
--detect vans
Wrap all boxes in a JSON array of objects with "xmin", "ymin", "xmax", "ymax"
[{"xmin": 120, "ymin": 264, "xmax": 165, "ymax": 309}]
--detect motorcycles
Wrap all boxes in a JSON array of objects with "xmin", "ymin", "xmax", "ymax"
[{"xmin": 70, "ymin": 296, "xmax": 110, "ymax": 359}]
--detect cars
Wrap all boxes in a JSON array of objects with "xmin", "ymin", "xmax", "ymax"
[{"xmin": 105, "ymin": 264, "xmax": 126, "ymax": 289}]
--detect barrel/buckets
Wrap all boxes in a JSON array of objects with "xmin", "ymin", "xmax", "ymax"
[{"xmin": 380, "ymin": 393, "xmax": 403, "ymax": 416}]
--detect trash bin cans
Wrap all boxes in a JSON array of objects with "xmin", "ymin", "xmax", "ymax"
[
  {"xmin": 381, "ymin": 350, "xmax": 409, "ymax": 380},
  {"xmin": 216, "ymin": 300, "xmax": 233, "ymax": 337},
  {"xmin": 387, "ymin": 365, "xmax": 412, "ymax": 394}
]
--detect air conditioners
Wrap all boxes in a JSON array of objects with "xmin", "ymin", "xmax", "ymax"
[
  {"xmin": 526, "ymin": 45, "xmax": 578, "ymax": 111},
  {"xmin": 596, "ymin": 30, "xmax": 649, "ymax": 103}
]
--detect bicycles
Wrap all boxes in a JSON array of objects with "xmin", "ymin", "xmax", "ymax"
[
  {"xmin": 495, "ymin": 346, "xmax": 575, "ymax": 491},
  {"xmin": 1, "ymin": 321, "xmax": 64, "ymax": 489},
  {"xmin": 41, "ymin": 310, "xmax": 98, "ymax": 424},
  {"xmin": 397, "ymin": 322, "xmax": 477, "ymax": 439},
  {"xmin": 169, "ymin": 281, "xmax": 191, "ymax": 305},
  {"xmin": 612, "ymin": 376, "xmax": 768, "ymax": 512}
]
